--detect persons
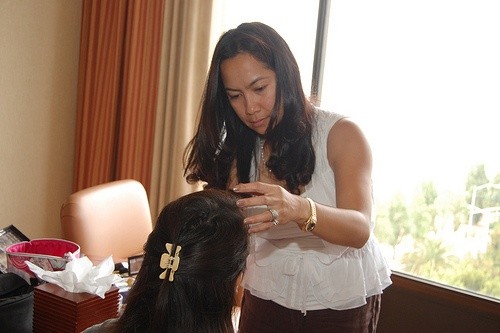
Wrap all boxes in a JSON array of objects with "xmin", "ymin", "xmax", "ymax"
[
  {"xmin": 181, "ymin": 21, "xmax": 393, "ymax": 333},
  {"xmin": 77, "ymin": 188, "xmax": 253, "ymax": 333}
]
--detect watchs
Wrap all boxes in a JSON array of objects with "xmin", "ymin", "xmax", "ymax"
[{"xmin": 297, "ymin": 197, "xmax": 318, "ymax": 232}]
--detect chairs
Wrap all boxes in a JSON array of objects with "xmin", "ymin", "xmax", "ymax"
[{"xmin": 60, "ymin": 179, "xmax": 153, "ymax": 267}]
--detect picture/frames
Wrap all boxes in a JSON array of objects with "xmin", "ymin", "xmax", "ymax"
[{"xmin": 128, "ymin": 254, "xmax": 145, "ymax": 275}]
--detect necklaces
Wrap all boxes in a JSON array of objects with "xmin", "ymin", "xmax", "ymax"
[{"xmin": 260, "ymin": 135, "xmax": 272, "ymax": 172}]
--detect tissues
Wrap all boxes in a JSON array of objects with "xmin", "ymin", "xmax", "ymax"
[{"xmin": 22, "ymin": 252, "xmax": 120, "ymax": 333}]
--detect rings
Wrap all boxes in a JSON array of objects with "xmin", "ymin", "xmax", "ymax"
[{"xmin": 267, "ymin": 209, "xmax": 279, "ymax": 230}]
